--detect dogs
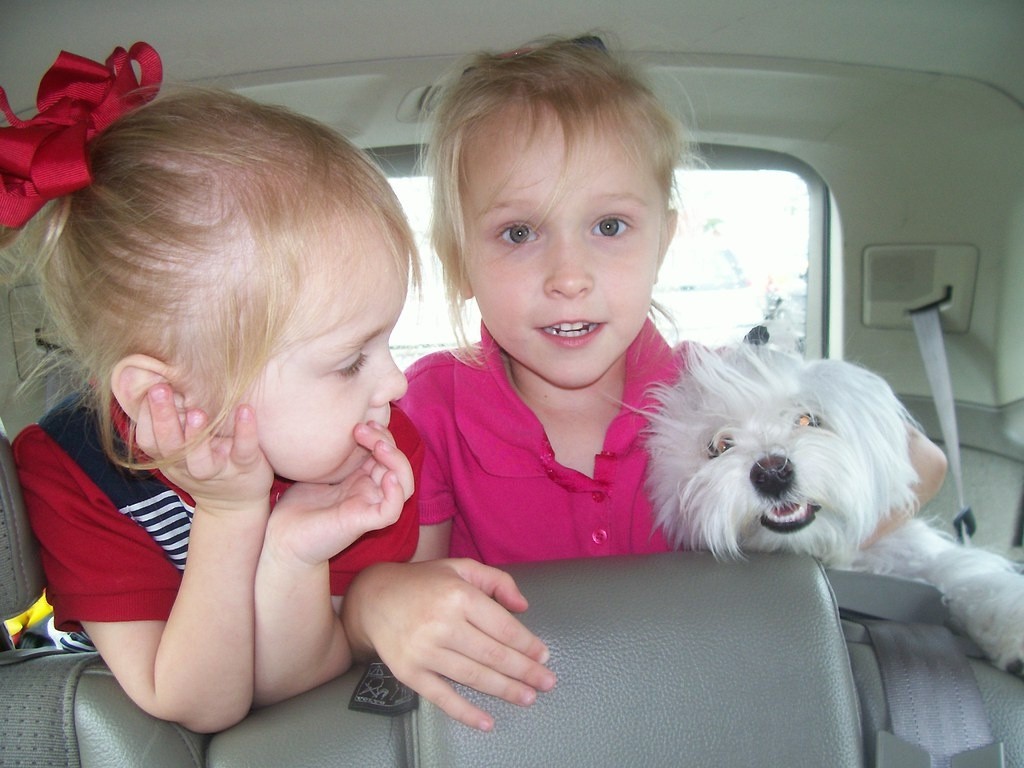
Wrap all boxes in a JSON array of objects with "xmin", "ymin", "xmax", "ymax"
[{"xmin": 646, "ymin": 346, "xmax": 1024, "ymax": 675}]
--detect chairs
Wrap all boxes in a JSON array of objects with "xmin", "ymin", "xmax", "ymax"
[{"xmin": 2, "ymin": 420, "xmax": 1024, "ymax": 768}]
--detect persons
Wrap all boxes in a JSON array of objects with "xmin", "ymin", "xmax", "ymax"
[
  {"xmin": 339, "ymin": 36, "xmax": 948, "ymax": 731},
  {"xmin": 12, "ymin": 92, "xmax": 428, "ymax": 733}
]
